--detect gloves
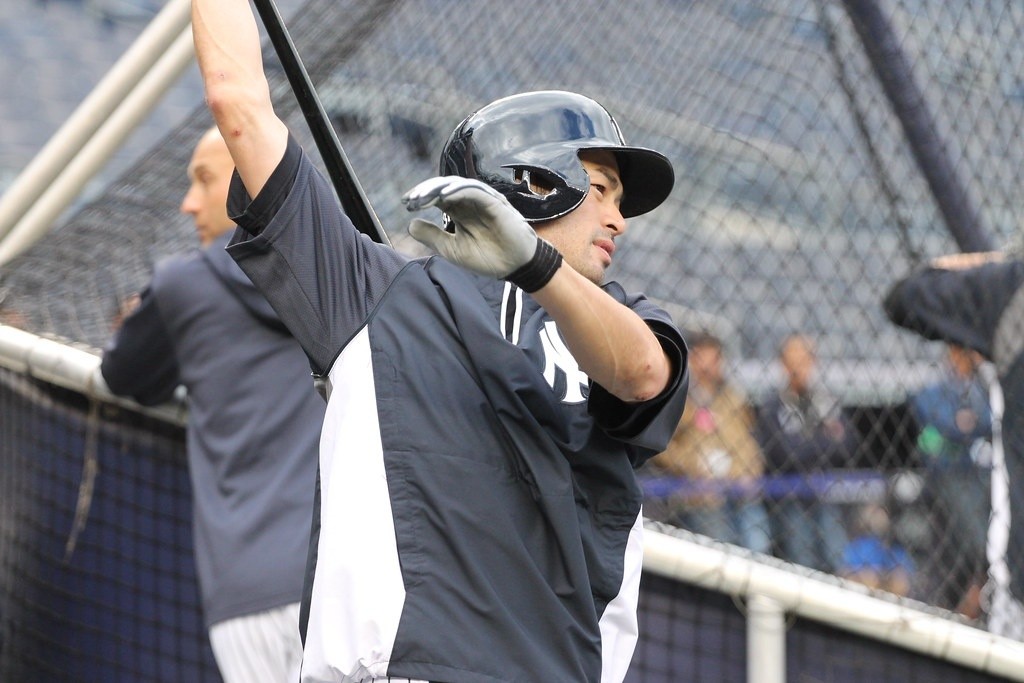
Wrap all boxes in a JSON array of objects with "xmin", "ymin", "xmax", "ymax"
[{"xmin": 401, "ymin": 175, "xmax": 562, "ymax": 293}]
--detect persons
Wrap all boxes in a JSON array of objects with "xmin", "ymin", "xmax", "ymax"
[
  {"xmin": 756, "ymin": 332, "xmax": 859, "ymax": 575},
  {"xmin": 99, "ymin": 120, "xmax": 326, "ymax": 683},
  {"xmin": 182, "ymin": 0, "xmax": 690, "ymax": 683},
  {"xmin": 658, "ymin": 332, "xmax": 770, "ymax": 561},
  {"xmin": 909, "ymin": 340, "xmax": 991, "ymax": 626},
  {"xmin": 884, "ymin": 246, "xmax": 1024, "ymax": 598},
  {"xmin": 841, "ymin": 504, "xmax": 925, "ymax": 598}
]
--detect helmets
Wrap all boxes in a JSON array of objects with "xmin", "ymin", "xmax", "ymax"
[{"xmin": 439, "ymin": 89, "xmax": 675, "ymax": 233}]
